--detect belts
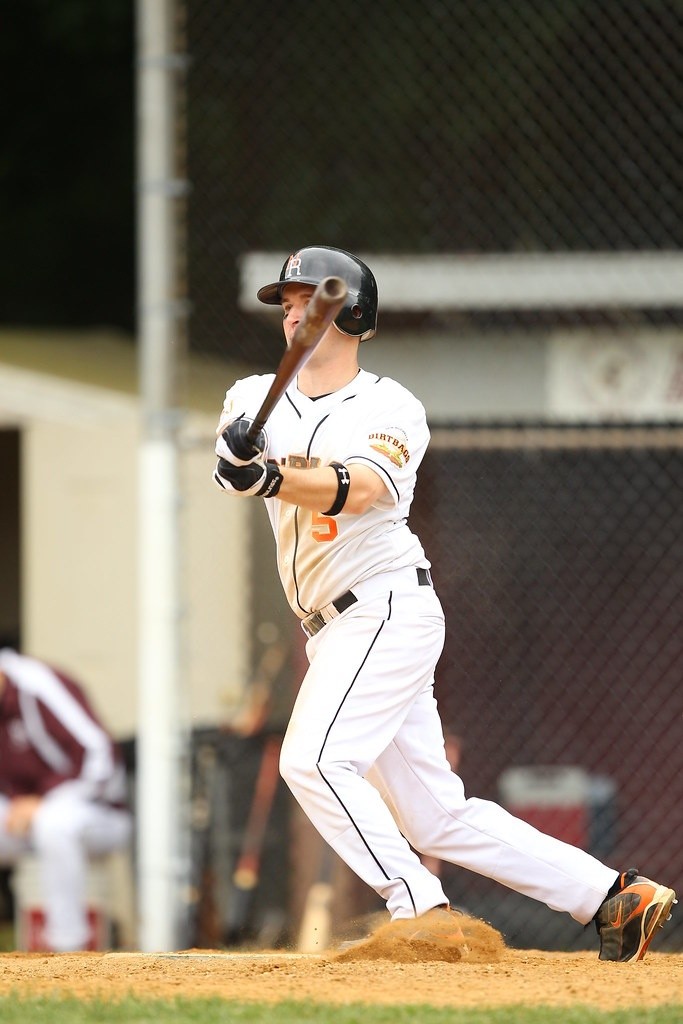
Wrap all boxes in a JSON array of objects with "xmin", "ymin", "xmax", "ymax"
[{"xmin": 301, "ymin": 569, "xmax": 430, "ymax": 636}]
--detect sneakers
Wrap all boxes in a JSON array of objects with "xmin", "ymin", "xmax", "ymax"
[
  {"xmin": 340, "ymin": 905, "xmax": 468, "ymax": 962},
  {"xmin": 593, "ymin": 868, "xmax": 677, "ymax": 964}
]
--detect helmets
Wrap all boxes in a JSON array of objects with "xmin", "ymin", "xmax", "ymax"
[{"xmin": 258, "ymin": 246, "xmax": 379, "ymax": 340}]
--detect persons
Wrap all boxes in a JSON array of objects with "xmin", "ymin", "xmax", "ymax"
[
  {"xmin": 0, "ymin": 648, "xmax": 137, "ymax": 954},
  {"xmin": 213, "ymin": 244, "xmax": 676, "ymax": 964}
]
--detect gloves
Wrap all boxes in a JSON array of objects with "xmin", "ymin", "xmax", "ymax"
[
  {"xmin": 215, "ymin": 412, "xmax": 269, "ymax": 468},
  {"xmin": 213, "ymin": 458, "xmax": 285, "ymax": 499}
]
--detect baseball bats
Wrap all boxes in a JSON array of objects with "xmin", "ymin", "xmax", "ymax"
[{"xmin": 244, "ymin": 274, "xmax": 350, "ymax": 448}]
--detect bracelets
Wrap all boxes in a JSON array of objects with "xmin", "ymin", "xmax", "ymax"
[{"xmin": 322, "ymin": 462, "xmax": 350, "ymax": 516}]
